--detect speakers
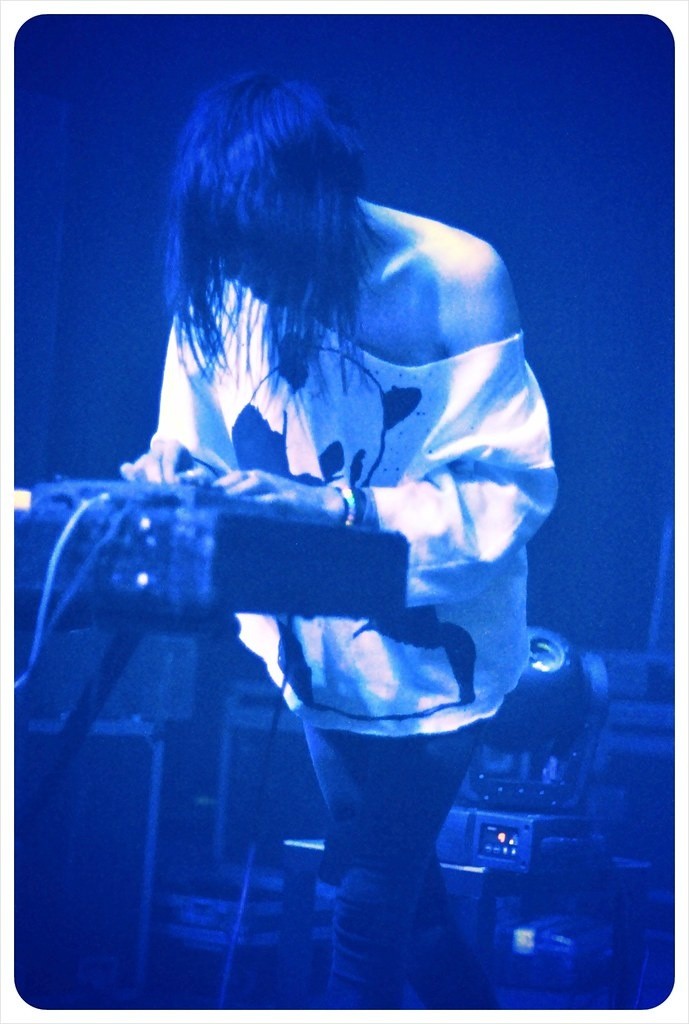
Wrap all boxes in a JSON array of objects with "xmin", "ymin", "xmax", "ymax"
[{"xmin": 213, "ymin": 688, "xmax": 335, "ymax": 872}]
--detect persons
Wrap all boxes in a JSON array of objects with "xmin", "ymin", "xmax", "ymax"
[{"xmin": 119, "ymin": 74, "xmax": 559, "ymax": 1009}]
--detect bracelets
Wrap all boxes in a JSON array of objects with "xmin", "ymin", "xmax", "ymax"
[{"xmin": 329, "ymin": 482, "xmax": 368, "ymax": 526}]
